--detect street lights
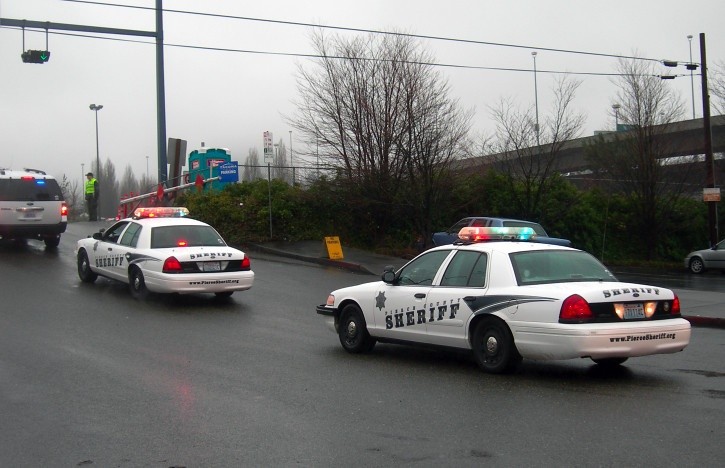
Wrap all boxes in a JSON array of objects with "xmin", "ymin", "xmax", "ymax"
[
  {"xmin": 289, "ymin": 129, "xmax": 293, "ymax": 165},
  {"xmin": 145, "ymin": 156, "xmax": 150, "ymax": 183},
  {"xmin": 531, "ymin": 51, "xmax": 541, "ymax": 148},
  {"xmin": 81, "ymin": 163, "xmax": 85, "ymax": 205},
  {"xmin": 611, "ymin": 104, "xmax": 622, "ymax": 131},
  {"xmin": 88, "ymin": 104, "xmax": 104, "ymax": 210},
  {"xmin": 688, "ymin": 34, "xmax": 697, "ymax": 119}
]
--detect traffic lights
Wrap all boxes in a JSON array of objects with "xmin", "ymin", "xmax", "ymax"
[{"xmin": 26, "ymin": 49, "xmax": 50, "ymax": 64}]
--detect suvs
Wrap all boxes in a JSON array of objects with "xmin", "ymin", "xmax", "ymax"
[{"xmin": 0, "ymin": 168, "xmax": 68, "ymax": 250}]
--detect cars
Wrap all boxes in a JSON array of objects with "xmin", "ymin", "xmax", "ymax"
[
  {"xmin": 428, "ymin": 217, "xmax": 575, "ymax": 252},
  {"xmin": 684, "ymin": 240, "xmax": 725, "ymax": 274},
  {"xmin": 72, "ymin": 206, "xmax": 255, "ymax": 301},
  {"xmin": 314, "ymin": 226, "xmax": 692, "ymax": 381}
]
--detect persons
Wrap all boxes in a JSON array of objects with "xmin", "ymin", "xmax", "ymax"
[{"xmin": 85, "ymin": 173, "xmax": 99, "ymax": 221}]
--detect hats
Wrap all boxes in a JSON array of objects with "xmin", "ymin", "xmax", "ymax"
[{"xmin": 85, "ymin": 173, "xmax": 92, "ymax": 176}]
